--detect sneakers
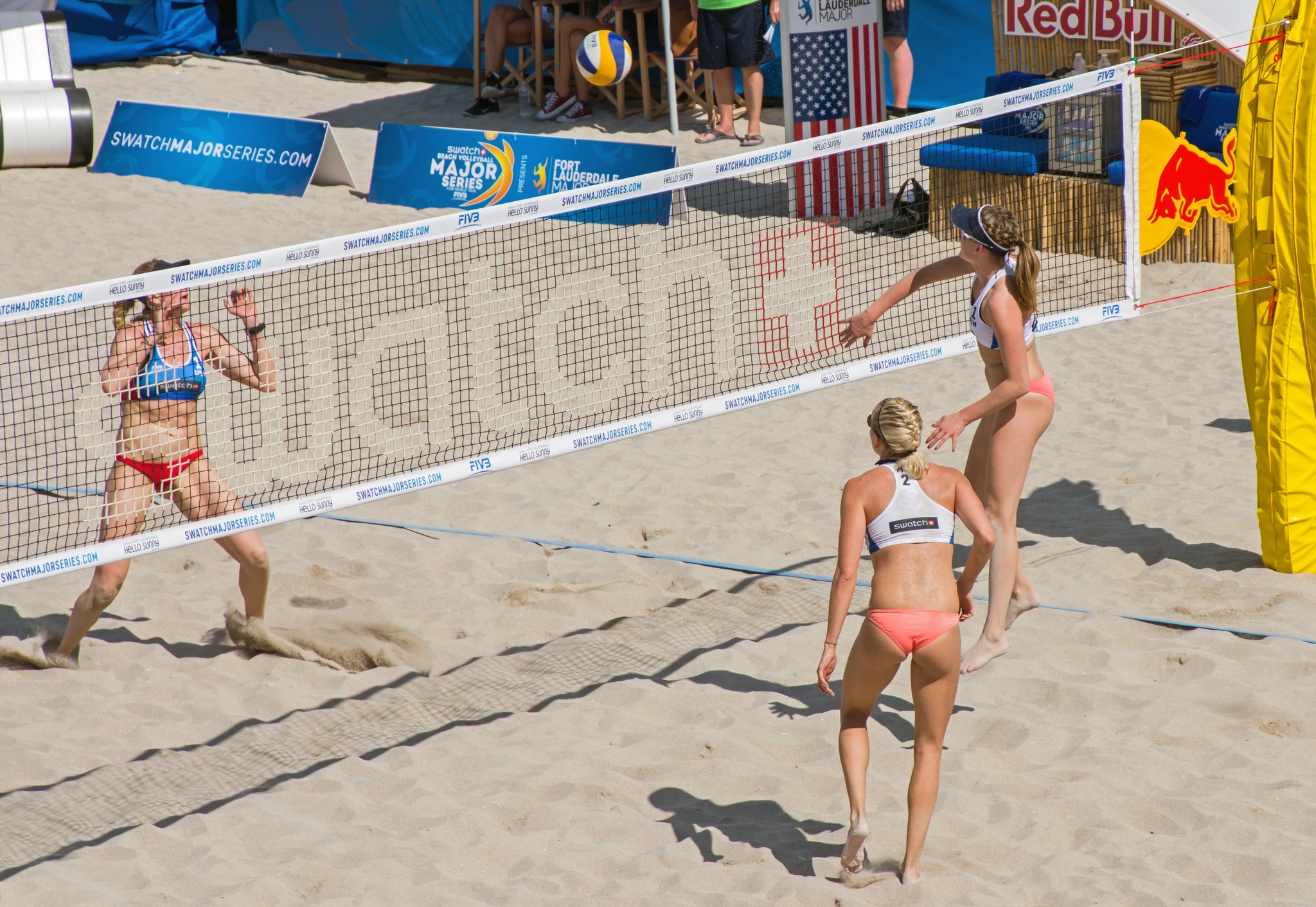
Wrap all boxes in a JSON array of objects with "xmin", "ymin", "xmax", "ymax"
[
  {"xmin": 536, "ymin": 88, "xmax": 576, "ymax": 119},
  {"xmin": 557, "ymin": 100, "xmax": 593, "ymax": 122},
  {"xmin": 463, "ymin": 96, "xmax": 500, "ymax": 118},
  {"xmin": 485, "ymin": 71, "xmax": 503, "ymax": 90}
]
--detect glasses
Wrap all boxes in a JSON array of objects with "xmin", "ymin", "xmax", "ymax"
[
  {"xmin": 867, "ymin": 415, "xmax": 881, "ymax": 439},
  {"xmin": 960, "ymin": 230, "xmax": 993, "ymax": 249}
]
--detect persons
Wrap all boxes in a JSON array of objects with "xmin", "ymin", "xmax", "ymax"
[
  {"xmin": 48, "ymin": 259, "xmax": 280, "ymax": 670},
  {"xmin": 844, "ymin": 203, "xmax": 1057, "ymax": 674},
  {"xmin": 690, "ymin": 0, "xmax": 783, "ymax": 147},
  {"xmin": 462, "ymin": 0, "xmax": 560, "ymax": 120},
  {"xmin": 536, "ymin": 0, "xmax": 663, "ymax": 123},
  {"xmin": 822, "ymin": 398, "xmax": 998, "ymax": 891},
  {"xmin": 877, "ymin": 0, "xmax": 915, "ymax": 121}
]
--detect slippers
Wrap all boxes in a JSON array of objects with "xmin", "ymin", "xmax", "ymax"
[
  {"xmin": 740, "ymin": 134, "xmax": 763, "ymax": 146},
  {"xmin": 694, "ymin": 129, "xmax": 739, "ymax": 144}
]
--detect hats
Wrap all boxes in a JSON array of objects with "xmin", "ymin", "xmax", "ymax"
[
  {"xmin": 951, "ymin": 204, "xmax": 1010, "ymax": 255},
  {"xmin": 139, "ymin": 259, "xmax": 191, "ymax": 303}
]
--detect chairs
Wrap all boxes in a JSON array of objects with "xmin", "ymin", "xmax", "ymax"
[
  {"xmin": 919, "ymin": 71, "xmax": 1054, "ymax": 175},
  {"xmin": 474, "ymin": 0, "xmax": 583, "ymax": 108},
  {"xmin": 548, "ymin": 0, "xmax": 661, "ymax": 119},
  {"xmin": 634, "ymin": 0, "xmax": 751, "ymax": 129},
  {"xmin": 1108, "ymin": 84, "xmax": 1240, "ymax": 191}
]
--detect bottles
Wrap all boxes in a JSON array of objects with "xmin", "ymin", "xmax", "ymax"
[
  {"xmin": 1073, "ymin": 53, "xmax": 1086, "ymax": 76},
  {"xmin": 1098, "ymin": 53, "xmax": 1111, "ymax": 94},
  {"xmin": 518, "ymin": 81, "xmax": 530, "ymax": 115},
  {"xmin": 879, "ymin": 224, "xmax": 891, "ymax": 234}
]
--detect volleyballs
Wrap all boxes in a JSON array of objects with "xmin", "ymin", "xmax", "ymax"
[{"xmin": 575, "ymin": 30, "xmax": 633, "ymax": 87}]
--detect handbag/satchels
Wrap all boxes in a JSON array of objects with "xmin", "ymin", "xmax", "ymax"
[
  {"xmin": 894, "ymin": 179, "xmax": 929, "ymax": 227},
  {"xmin": 858, "ymin": 177, "xmax": 929, "ymax": 239}
]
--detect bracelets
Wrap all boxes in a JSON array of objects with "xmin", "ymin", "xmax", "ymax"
[{"xmin": 244, "ymin": 323, "xmax": 265, "ymax": 336}]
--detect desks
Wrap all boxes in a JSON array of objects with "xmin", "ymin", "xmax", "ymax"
[{"xmin": 1086, "ymin": 54, "xmax": 1220, "ymax": 139}]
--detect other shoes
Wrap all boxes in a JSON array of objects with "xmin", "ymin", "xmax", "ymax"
[{"xmin": 887, "ymin": 114, "xmax": 908, "ymax": 121}]
